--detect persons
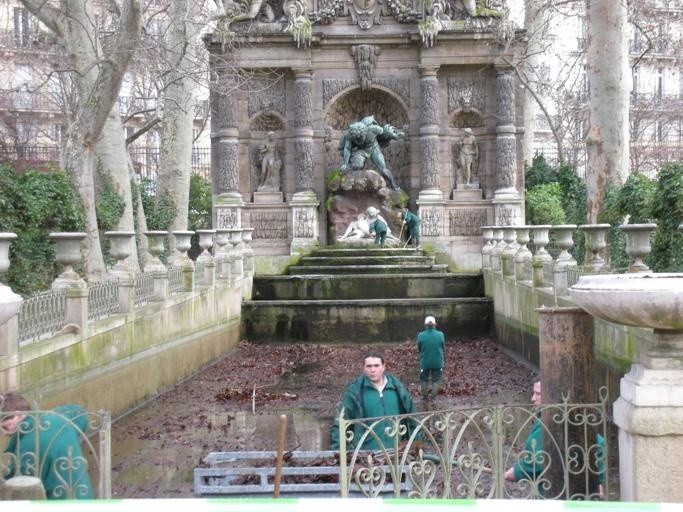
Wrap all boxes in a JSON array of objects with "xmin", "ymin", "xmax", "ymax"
[
  {"xmin": 256, "ymin": 131, "xmax": 282, "ymax": 191},
  {"xmin": 0, "ymin": 390, "xmax": 95, "ymax": 499},
  {"xmin": 394, "ymin": 208, "xmax": 420, "ymax": 249},
  {"xmin": 335, "ymin": 114, "xmax": 404, "ymax": 192},
  {"xmin": 496, "ymin": 375, "xmax": 607, "ymax": 500},
  {"xmin": 334, "ymin": 206, "xmax": 391, "ymax": 243},
  {"xmin": 453, "ymin": 127, "xmax": 479, "ymax": 185},
  {"xmin": 416, "ymin": 315, "xmax": 445, "ymax": 412},
  {"xmin": 367, "ymin": 215, "xmax": 386, "ymax": 248},
  {"xmin": 328, "ymin": 353, "xmax": 424, "ymax": 483}
]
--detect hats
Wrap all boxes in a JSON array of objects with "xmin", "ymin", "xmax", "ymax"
[{"xmin": 424, "ymin": 316, "xmax": 435, "ymax": 324}]
